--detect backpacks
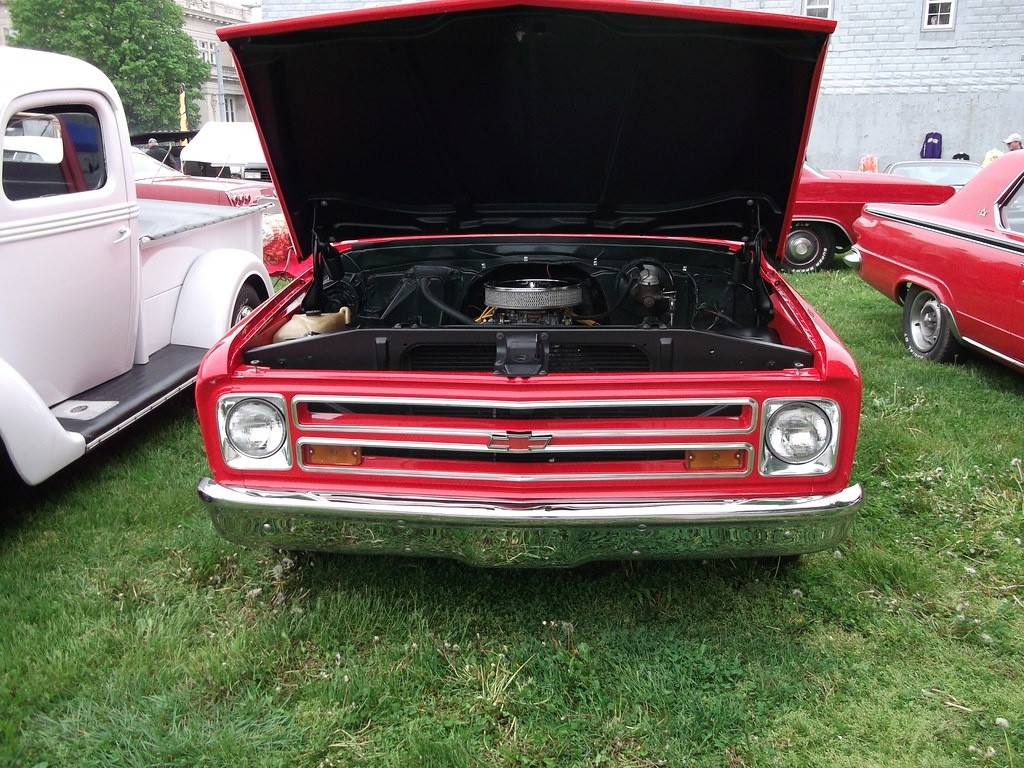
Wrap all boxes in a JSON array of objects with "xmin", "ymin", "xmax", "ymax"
[
  {"xmin": 920, "ymin": 132, "xmax": 943, "ymax": 158},
  {"xmin": 982, "ymin": 149, "xmax": 1003, "ymax": 168}
]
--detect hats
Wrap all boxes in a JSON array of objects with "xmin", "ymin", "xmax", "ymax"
[
  {"xmin": 1002, "ymin": 133, "xmax": 1021, "ymax": 143},
  {"xmin": 148, "ymin": 138, "xmax": 157, "ymax": 143}
]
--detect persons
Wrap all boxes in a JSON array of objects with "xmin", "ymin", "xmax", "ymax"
[
  {"xmin": 1002, "ymin": 133, "xmax": 1024, "ymax": 151},
  {"xmin": 146, "ymin": 138, "xmax": 171, "ymax": 167}
]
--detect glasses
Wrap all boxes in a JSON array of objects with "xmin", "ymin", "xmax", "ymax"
[{"xmin": 1008, "ymin": 142, "xmax": 1013, "ymax": 145}]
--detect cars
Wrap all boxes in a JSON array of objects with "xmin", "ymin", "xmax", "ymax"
[
  {"xmin": 780, "ymin": 156, "xmax": 958, "ymax": 275},
  {"xmin": 194, "ymin": 0, "xmax": 860, "ymax": 562},
  {"xmin": 0, "ymin": 45, "xmax": 274, "ymax": 485},
  {"xmin": 179, "ymin": 119, "xmax": 272, "ymax": 191},
  {"xmin": 857, "ymin": 149, "xmax": 1024, "ymax": 372},
  {"xmin": 880, "ymin": 157, "xmax": 1022, "ymax": 213}
]
